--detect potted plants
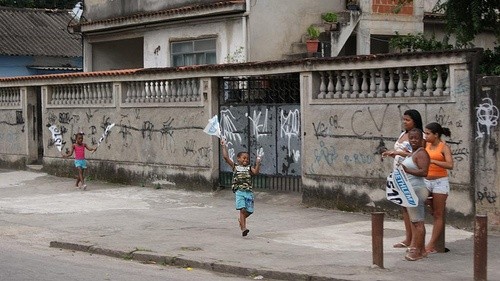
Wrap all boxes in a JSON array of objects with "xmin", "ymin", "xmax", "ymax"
[
  {"xmin": 305, "ymin": 25, "xmax": 320, "ymax": 53},
  {"xmin": 321, "ymin": 12, "xmax": 338, "ymax": 31}
]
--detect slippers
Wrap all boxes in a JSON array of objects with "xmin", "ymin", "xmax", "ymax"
[
  {"xmin": 406, "ymin": 247, "xmax": 416, "ymax": 253},
  {"xmin": 393, "ymin": 242, "xmax": 407, "ymax": 248}
]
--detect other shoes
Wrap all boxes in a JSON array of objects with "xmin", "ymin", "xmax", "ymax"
[
  {"xmin": 82, "ymin": 185, "xmax": 87, "ymax": 191},
  {"xmin": 243, "ymin": 229, "xmax": 249, "ymax": 236},
  {"xmin": 426, "ymin": 249, "xmax": 437, "ymax": 253},
  {"xmin": 406, "ymin": 254, "xmax": 423, "ymax": 260}
]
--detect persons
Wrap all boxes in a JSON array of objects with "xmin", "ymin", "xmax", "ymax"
[
  {"xmin": 382, "ymin": 110, "xmax": 453, "ymax": 261},
  {"xmin": 63, "ymin": 133, "xmax": 97, "ymax": 190},
  {"xmin": 219, "ymin": 140, "xmax": 261, "ymax": 235}
]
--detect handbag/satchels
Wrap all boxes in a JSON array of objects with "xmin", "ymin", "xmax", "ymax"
[{"xmin": 385, "ymin": 164, "xmax": 418, "ymax": 207}]
